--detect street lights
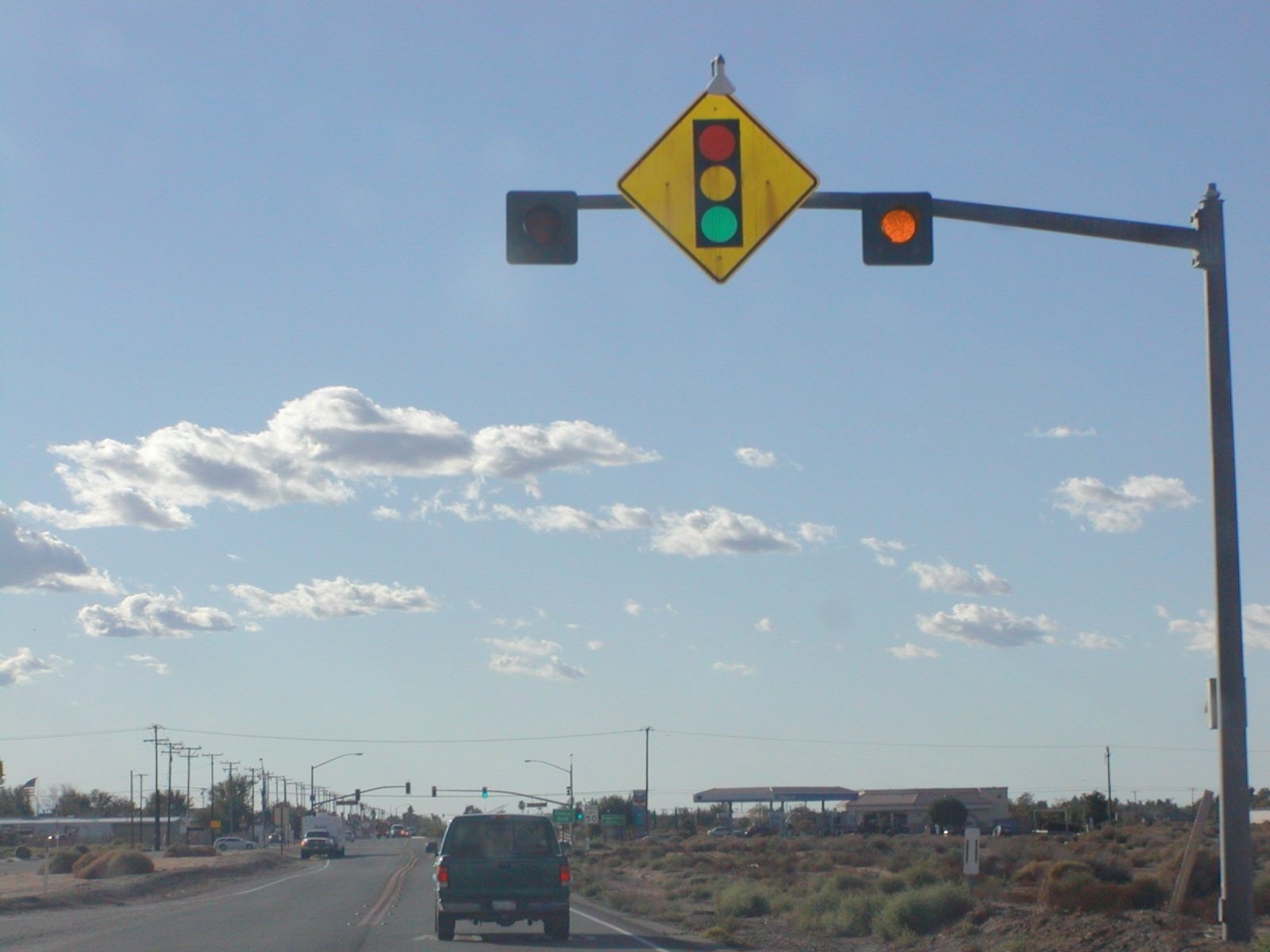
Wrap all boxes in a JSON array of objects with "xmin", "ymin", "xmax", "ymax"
[
  {"xmin": 524, "ymin": 753, "xmax": 573, "ymax": 810},
  {"xmin": 310, "ymin": 752, "xmax": 362, "ymax": 814}
]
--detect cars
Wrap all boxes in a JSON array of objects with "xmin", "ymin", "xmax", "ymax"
[
  {"xmin": 943, "ymin": 826, "xmax": 954, "ymax": 834},
  {"xmin": 267, "ymin": 832, "xmax": 282, "ymax": 843},
  {"xmin": 405, "ymin": 827, "xmax": 418, "ymax": 837},
  {"xmin": 299, "ymin": 830, "xmax": 343, "ymax": 859},
  {"xmin": 707, "ymin": 826, "xmax": 742, "ymax": 837},
  {"xmin": 342, "ymin": 831, "xmax": 355, "ymax": 842},
  {"xmin": 213, "ymin": 836, "xmax": 257, "ymax": 853}
]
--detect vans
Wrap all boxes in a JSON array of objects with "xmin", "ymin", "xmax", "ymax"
[{"xmin": 391, "ymin": 824, "xmax": 405, "ymax": 838}]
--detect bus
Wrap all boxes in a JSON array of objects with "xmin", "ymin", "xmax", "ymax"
[{"xmin": 374, "ymin": 821, "xmax": 390, "ymax": 839}]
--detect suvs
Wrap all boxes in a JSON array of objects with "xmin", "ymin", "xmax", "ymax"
[{"xmin": 744, "ymin": 826, "xmax": 768, "ymax": 837}]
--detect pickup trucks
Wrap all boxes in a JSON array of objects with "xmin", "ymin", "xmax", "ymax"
[{"xmin": 426, "ymin": 813, "xmax": 573, "ymax": 940}]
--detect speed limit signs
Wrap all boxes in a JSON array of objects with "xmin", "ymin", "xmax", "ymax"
[{"xmin": 584, "ymin": 806, "xmax": 599, "ymax": 824}]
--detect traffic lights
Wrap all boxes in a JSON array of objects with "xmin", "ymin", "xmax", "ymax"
[
  {"xmin": 575, "ymin": 808, "xmax": 583, "ymax": 820},
  {"xmin": 432, "ymin": 786, "xmax": 437, "ymax": 797},
  {"xmin": 505, "ymin": 189, "xmax": 578, "ymax": 267},
  {"xmin": 861, "ymin": 191, "xmax": 934, "ymax": 267},
  {"xmin": 482, "ymin": 787, "xmax": 488, "ymax": 798}
]
